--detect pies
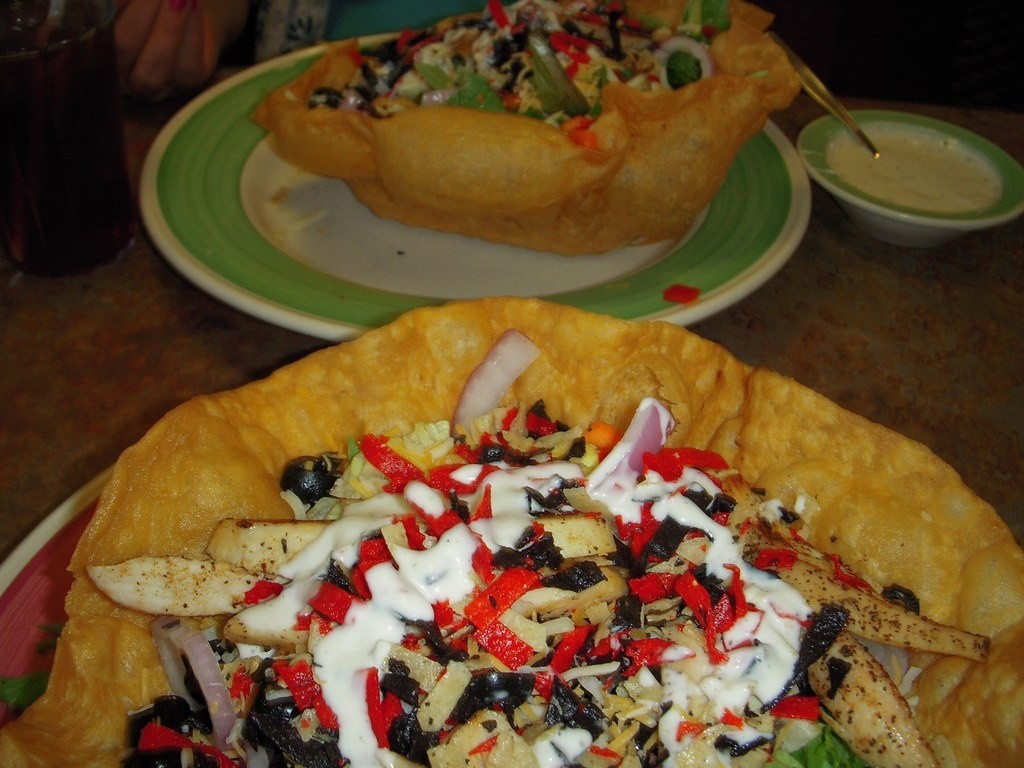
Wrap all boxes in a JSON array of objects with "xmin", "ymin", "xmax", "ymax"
[
  {"xmin": 244, "ymin": 0, "xmax": 810, "ymax": 256},
  {"xmin": 0, "ymin": 294, "xmax": 1024, "ymax": 768}
]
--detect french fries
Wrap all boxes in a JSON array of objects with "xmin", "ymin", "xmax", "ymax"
[{"xmin": 80, "ymin": 514, "xmax": 627, "ymax": 649}]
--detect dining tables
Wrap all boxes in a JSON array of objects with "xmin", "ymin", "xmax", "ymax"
[{"xmin": 0, "ymin": 69, "xmax": 1024, "ymax": 768}]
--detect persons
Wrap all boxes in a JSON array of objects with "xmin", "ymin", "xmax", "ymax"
[{"xmin": 36, "ymin": 0, "xmax": 523, "ymax": 99}]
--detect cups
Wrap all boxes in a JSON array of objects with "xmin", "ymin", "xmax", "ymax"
[{"xmin": 0, "ymin": 0, "xmax": 132, "ymax": 276}]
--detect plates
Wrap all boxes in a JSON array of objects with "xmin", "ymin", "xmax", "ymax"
[
  {"xmin": 0, "ymin": 463, "xmax": 114, "ymax": 731},
  {"xmin": 139, "ymin": 27, "xmax": 811, "ymax": 342}
]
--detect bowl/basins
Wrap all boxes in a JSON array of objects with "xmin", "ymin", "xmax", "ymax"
[{"xmin": 797, "ymin": 109, "xmax": 1024, "ymax": 248}]
